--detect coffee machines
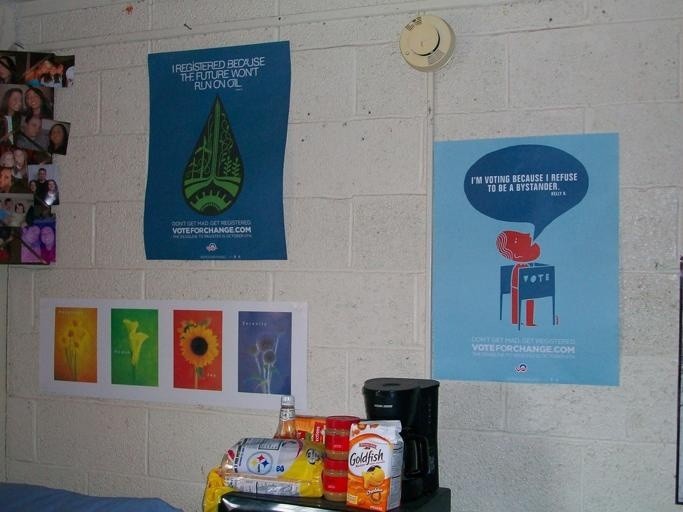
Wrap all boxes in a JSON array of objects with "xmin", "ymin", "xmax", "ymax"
[{"xmin": 362, "ymin": 377, "xmax": 440, "ymax": 508}]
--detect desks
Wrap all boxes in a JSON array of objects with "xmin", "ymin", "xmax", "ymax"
[{"xmin": 220, "ymin": 486, "xmax": 451, "ymax": 511}]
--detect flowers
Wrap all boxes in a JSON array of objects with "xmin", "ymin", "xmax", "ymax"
[{"xmin": 57, "ymin": 318, "xmax": 280, "ymax": 394}]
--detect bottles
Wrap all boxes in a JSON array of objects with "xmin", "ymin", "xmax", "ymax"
[{"xmin": 274, "ymin": 395, "xmax": 298, "ymax": 441}]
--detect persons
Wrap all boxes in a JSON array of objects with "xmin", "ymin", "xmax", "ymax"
[{"xmin": 0, "ymin": 50, "xmax": 75, "ymax": 266}]
